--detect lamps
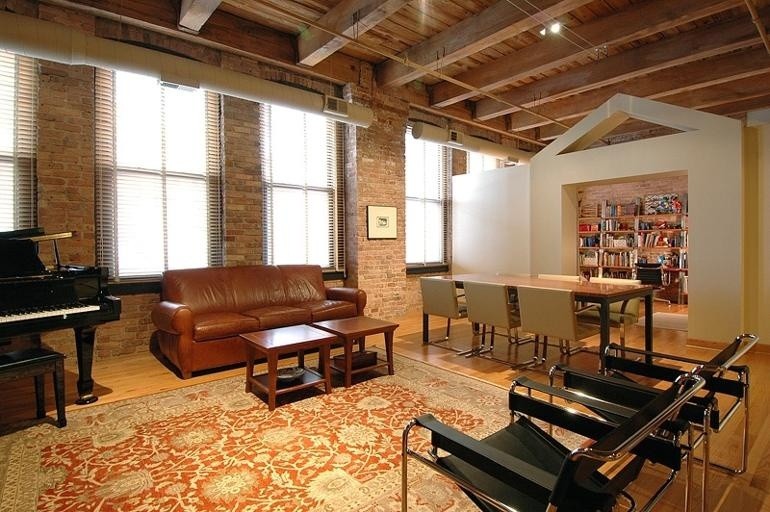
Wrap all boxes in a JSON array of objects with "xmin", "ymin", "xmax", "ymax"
[{"xmin": 505, "ymin": 0, "xmax": 609, "ymax": 60}]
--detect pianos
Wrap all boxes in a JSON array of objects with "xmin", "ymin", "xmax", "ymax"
[{"xmin": 0, "ymin": 228, "xmax": 121, "ymax": 405}]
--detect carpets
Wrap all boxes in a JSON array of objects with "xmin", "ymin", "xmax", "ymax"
[{"xmin": 0, "ymin": 343, "xmax": 603, "ymax": 512}]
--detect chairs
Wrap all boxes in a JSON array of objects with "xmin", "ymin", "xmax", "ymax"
[
  {"xmin": 677, "ymin": 277, "xmax": 688, "ymax": 305},
  {"xmin": 548, "ymin": 334, "xmax": 761, "ymax": 512},
  {"xmin": 419, "ymin": 271, "xmax": 653, "ymax": 371},
  {"xmin": 634, "ymin": 263, "xmax": 671, "ymax": 305},
  {"xmin": 401, "ymin": 374, "xmax": 706, "ymax": 512}
]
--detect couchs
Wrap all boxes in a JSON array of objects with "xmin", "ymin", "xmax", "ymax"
[{"xmin": 151, "ymin": 265, "xmax": 367, "ymax": 380}]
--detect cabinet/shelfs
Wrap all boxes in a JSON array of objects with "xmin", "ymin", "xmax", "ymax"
[{"xmin": 577, "ymin": 193, "xmax": 688, "ymax": 286}]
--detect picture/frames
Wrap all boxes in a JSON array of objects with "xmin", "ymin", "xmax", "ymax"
[{"xmin": 366, "ymin": 205, "xmax": 398, "ymax": 240}]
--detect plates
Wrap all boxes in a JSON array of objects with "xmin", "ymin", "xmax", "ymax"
[{"xmin": 277, "ymin": 367, "xmax": 305, "ymax": 382}]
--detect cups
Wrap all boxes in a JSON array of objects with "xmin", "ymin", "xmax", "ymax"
[{"xmin": 496, "ymin": 272, "xmax": 504, "ymax": 281}]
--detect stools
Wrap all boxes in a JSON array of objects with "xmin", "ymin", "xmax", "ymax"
[{"xmin": 0, "ymin": 346, "xmax": 68, "ymax": 428}]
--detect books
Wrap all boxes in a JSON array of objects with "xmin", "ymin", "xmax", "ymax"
[
  {"xmin": 630, "ymin": 243, "xmax": 632, "ymax": 244},
  {"xmin": 579, "ymin": 250, "xmax": 599, "ymax": 266},
  {"xmin": 606, "ymin": 200, "xmax": 636, "ymax": 217},
  {"xmin": 580, "ymin": 267, "xmax": 599, "ymax": 279},
  {"xmin": 640, "ymin": 232, "xmax": 684, "ymax": 248},
  {"xmin": 604, "ymin": 218, "xmax": 637, "ymax": 232},
  {"xmin": 604, "ymin": 269, "xmax": 633, "ymax": 280},
  {"xmin": 603, "ymin": 233, "xmax": 636, "ymax": 249}
]
